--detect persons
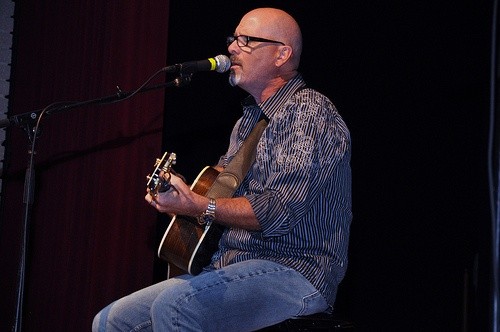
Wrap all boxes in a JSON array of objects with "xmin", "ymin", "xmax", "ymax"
[{"xmin": 88, "ymin": 8, "xmax": 352, "ymax": 332}]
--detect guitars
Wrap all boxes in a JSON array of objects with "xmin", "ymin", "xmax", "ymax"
[{"xmin": 144, "ymin": 148, "xmax": 234, "ymax": 278}]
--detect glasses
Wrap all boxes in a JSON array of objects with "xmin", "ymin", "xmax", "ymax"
[{"xmin": 225, "ymin": 34, "xmax": 285, "ymax": 47}]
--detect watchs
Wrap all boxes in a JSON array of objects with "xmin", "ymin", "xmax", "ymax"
[{"xmin": 201, "ymin": 198, "xmax": 216, "ymax": 223}]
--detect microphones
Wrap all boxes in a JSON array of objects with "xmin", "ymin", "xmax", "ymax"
[{"xmin": 162, "ymin": 55, "xmax": 231, "ymax": 72}]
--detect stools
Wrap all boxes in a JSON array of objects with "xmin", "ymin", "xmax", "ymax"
[{"xmin": 262, "ymin": 309, "xmax": 344, "ymax": 332}]
